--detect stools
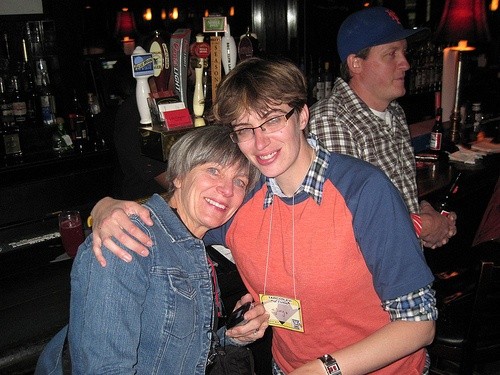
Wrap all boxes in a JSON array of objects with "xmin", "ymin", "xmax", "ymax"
[{"xmin": 449, "ymin": 239, "xmax": 500, "ymax": 349}]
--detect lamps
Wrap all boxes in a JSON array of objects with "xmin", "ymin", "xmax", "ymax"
[{"xmin": 450, "ymin": 40, "xmax": 477, "ymax": 133}]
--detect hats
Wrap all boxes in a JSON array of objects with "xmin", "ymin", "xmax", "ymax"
[{"xmin": 337, "ymin": 7, "xmax": 432, "ymax": 64}]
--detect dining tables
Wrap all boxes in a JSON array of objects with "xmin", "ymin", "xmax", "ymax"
[
  {"xmin": 0, "ymin": 199, "xmax": 103, "ymax": 371},
  {"xmin": 415, "ymin": 112, "xmax": 500, "ymax": 253}
]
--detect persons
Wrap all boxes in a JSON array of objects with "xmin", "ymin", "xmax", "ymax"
[
  {"xmin": 84, "ymin": 59, "xmax": 438, "ymax": 375},
  {"xmin": 317, "ymin": 353, "xmax": 342, "ymax": 375},
  {"xmin": 32, "ymin": 125, "xmax": 269, "ymax": 375},
  {"xmin": 304, "ymin": 9, "xmax": 457, "ymax": 375}
]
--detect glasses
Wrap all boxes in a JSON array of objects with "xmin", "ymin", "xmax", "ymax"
[{"xmin": 229, "ymin": 107, "xmax": 297, "ymax": 144}]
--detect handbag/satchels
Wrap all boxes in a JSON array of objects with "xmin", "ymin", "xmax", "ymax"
[{"xmin": 207, "ymin": 346, "xmax": 256, "ymax": 375}]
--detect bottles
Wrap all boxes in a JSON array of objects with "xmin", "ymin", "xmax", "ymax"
[
  {"xmin": 430, "ymin": 108, "xmax": 444, "ymax": 153},
  {"xmin": 1, "ymin": 19, "xmax": 97, "ymax": 160},
  {"xmin": 471, "ymin": 104, "xmax": 482, "ymax": 137}
]
